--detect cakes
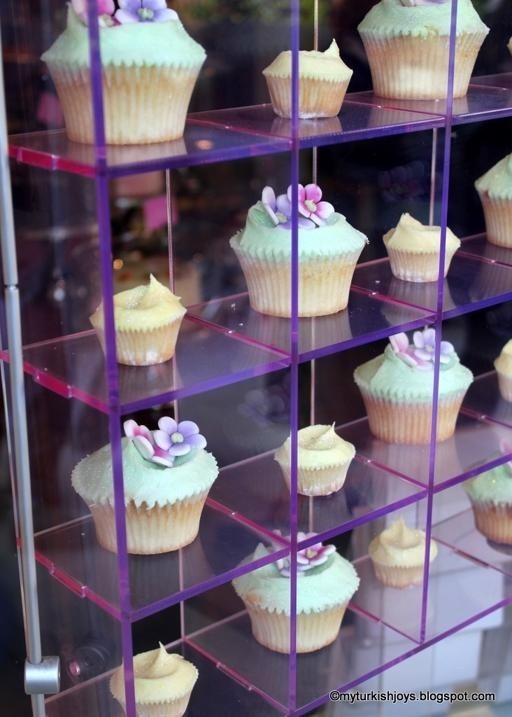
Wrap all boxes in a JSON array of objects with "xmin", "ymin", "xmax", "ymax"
[
  {"xmin": 273, "ymin": 421, "xmax": 355, "ymax": 499},
  {"xmin": 494, "ymin": 339, "xmax": 512, "ymax": 404},
  {"xmin": 41, "ymin": 0, "xmax": 208, "ymax": 145},
  {"xmin": 261, "ymin": 38, "xmax": 354, "ymax": 120},
  {"xmin": 229, "ymin": 183, "xmax": 370, "ymax": 319},
  {"xmin": 89, "ymin": 273, "xmax": 187, "ymax": 367},
  {"xmin": 70, "ymin": 417, "xmax": 219, "ymax": 554},
  {"xmin": 462, "ymin": 439, "xmax": 512, "ymax": 544},
  {"xmin": 368, "ymin": 518, "xmax": 438, "ymax": 588},
  {"xmin": 382, "ymin": 211, "xmax": 462, "ymax": 283},
  {"xmin": 352, "ymin": 324, "xmax": 474, "ymax": 446},
  {"xmin": 110, "ymin": 641, "xmax": 200, "ymax": 717},
  {"xmin": 473, "ymin": 152, "xmax": 512, "ymax": 249},
  {"xmin": 233, "ymin": 528, "xmax": 359, "ymax": 654},
  {"xmin": 357, "ymin": 0, "xmax": 490, "ymax": 101}
]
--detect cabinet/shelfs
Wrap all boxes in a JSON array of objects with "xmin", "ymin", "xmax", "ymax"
[{"xmin": 0, "ymin": 0, "xmax": 511, "ymax": 716}]
